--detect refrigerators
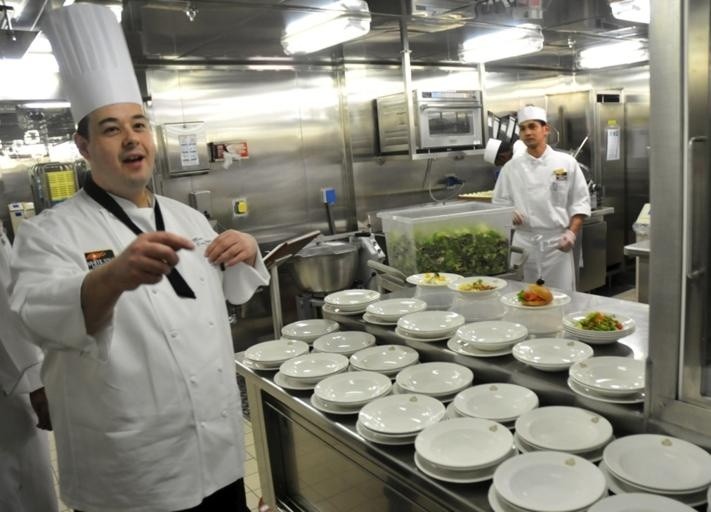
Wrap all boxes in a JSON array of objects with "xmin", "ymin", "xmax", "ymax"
[{"xmin": 546, "ymin": 89, "xmax": 649, "ymax": 276}]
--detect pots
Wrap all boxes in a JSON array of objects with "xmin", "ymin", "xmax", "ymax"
[{"xmin": 284, "ymin": 240, "xmax": 359, "ymax": 293}]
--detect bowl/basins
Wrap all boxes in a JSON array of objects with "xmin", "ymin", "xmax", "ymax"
[
  {"xmin": 411, "ymin": 287, "xmax": 455, "ymax": 311},
  {"xmin": 503, "ymin": 309, "xmax": 565, "ymax": 333},
  {"xmin": 447, "ymin": 298, "xmax": 507, "ymax": 320}
]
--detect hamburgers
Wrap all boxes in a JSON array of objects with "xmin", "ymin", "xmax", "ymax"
[{"xmin": 519, "ymin": 283, "xmax": 553, "ymax": 306}]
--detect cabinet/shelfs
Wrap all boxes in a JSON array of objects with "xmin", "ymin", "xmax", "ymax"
[
  {"xmin": 575, "ymin": 205, "xmax": 614, "ymax": 293},
  {"xmin": 234, "ymin": 276, "xmax": 711, "ymax": 512}
]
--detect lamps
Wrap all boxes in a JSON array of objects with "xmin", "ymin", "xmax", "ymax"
[{"xmin": 280, "ymin": 15, "xmax": 372, "ymax": 56}]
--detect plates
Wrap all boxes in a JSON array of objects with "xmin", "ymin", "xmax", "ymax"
[
  {"xmin": 273, "ymin": 352, "xmax": 349, "ymax": 390},
  {"xmin": 414, "ymin": 418, "xmax": 520, "ymax": 484},
  {"xmin": 356, "ymin": 393, "xmax": 447, "ymax": 447},
  {"xmin": 600, "ymin": 433, "xmax": 711, "ymax": 507},
  {"xmin": 406, "ymin": 273, "xmax": 464, "ymax": 288},
  {"xmin": 348, "ymin": 345, "xmax": 420, "ymax": 380},
  {"xmin": 311, "ymin": 331, "xmax": 376, "ymax": 359},
  {"xmin": 447, "ymin": 276, "xmax": 507, "ymax": 297},
  {"xmin": 511, "ymin": 337, "xmax": 594, "ymax": 372},
  {"xmin": 501, "ymin": 290, "xmax": 571, "ymax": 311},
  {"xmin": 242, "ymin": 339, "xmax": 309, "ymax": 371},
  {"xmin": 311, "ymin": 372, "xmax": 392, "ymax": 414},
  {"xmin": 448, "ymin": 383, "xmax": 539, "ymax": 429},
  {"xmin": 588, "ymin": 493, "xmax": 700, "ymax": 511},
  {"xmin": 560, "ymin": 311, "xmax": 636, "ymax": 344},
  {"xmin": 568, "ymin": 357, "xmax": 647, "ymax": 403},
  {"xmin": 395, "ymin": 309, "xmax": 465, "ymax": 342},
  {"xmin": 447, "ymin": 321, "xmax": 528, "ymax": 358},
  {"xmin": 281, "ymin": 319, "xmax": 340, "ymax": 347},
  {"xmin": 362, "ymin": 297, "xmax": 427, "ymax": 326},
  {"xmin": 322, "ymin": 288, "xmax": 381, "ymax": 315},
  {"xmin": 706, "ymin": 486, "xmax": 711, "ymax": 512},
  {"xmin": 515, "ymin": 405, "xmax": 617, "ymax": 462},
  {"xmin": 488, "ymin": 450, "xmax": 609, "ymax": 512},
  {"xmin": 392, "ymin": 362, "xmax": 474, "ymax": 403}
]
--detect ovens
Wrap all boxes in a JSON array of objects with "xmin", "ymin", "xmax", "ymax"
[{"xmin": 378, "ymin": 91, "xmax": 482, "ymax": 152}]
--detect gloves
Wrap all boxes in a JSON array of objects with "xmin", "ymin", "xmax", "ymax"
[
  {"xmin": 512, "ymin": 209, "xmax": 525, "ymax": 225},
  {"xmin": 558, "ymin": 229, "xmax": 576, "ymax": 253}
]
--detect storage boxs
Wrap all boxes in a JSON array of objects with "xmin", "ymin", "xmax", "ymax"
[{"xmin": 376, "ymin": 202, "xmax": 515, "ymax": 277}]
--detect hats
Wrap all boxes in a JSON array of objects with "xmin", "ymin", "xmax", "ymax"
[
  {"xmin": 39, "ymin": 3, "xmax": 144, "ymax": 123},
  {"xmin": 517, "ymin": 106, "xmax": 547, "ymax": 125},
  {"xmin": 484, "ymin": 138, "xmax": 502, "ymax": 165}
]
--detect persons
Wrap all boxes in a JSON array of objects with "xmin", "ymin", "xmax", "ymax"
[
  {"xmin": 480, "ymin": 136, "xmax": 586, "ymax": 268},
  {"xmin": 7, "ymin": 2, "xmax": 270, "ymax": 512},
  {"xmin": 492, "ymin": 106, "xmax": 591, "ymax": 292},
  {"xmin": 0, "ymin": 233, "xmax": 59, "ymax": 512}
]
